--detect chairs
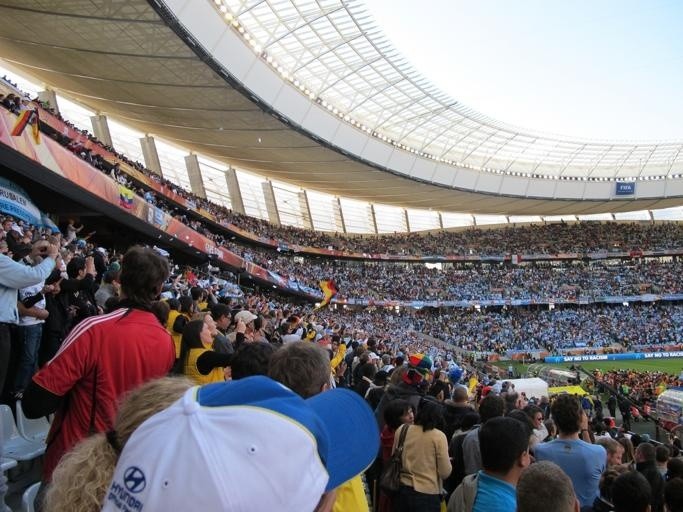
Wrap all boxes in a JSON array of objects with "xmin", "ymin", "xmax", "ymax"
[{"xmin": 0, "ymin": 395, "xmax": 62, "ymax": 512}]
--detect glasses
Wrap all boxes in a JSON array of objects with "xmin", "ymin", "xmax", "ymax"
[
  {"xmin": 227, "ymin": 316, "xmax": 233, "ymax": 321},
  {"xmin": 538, "ymin": 417, "xmax": 544, "ymax": 421}
]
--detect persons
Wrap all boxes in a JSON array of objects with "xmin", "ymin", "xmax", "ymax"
[{"xmin": 1, "ymin": 75, "xmax": 682, "ymax": 512}]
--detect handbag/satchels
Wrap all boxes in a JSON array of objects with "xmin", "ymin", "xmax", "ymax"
[{"xmin": 379, "ymin": 422, "xmax": 409, "ymax": 496}]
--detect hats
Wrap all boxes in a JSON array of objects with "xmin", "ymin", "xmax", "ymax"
[
  {"xmin": 100, "ymin": 376, "xmax": 381, "ymax": 512},
  {"xmin": 234, "ymin": 310, "xmax": 258, "ymax": 325}
]
